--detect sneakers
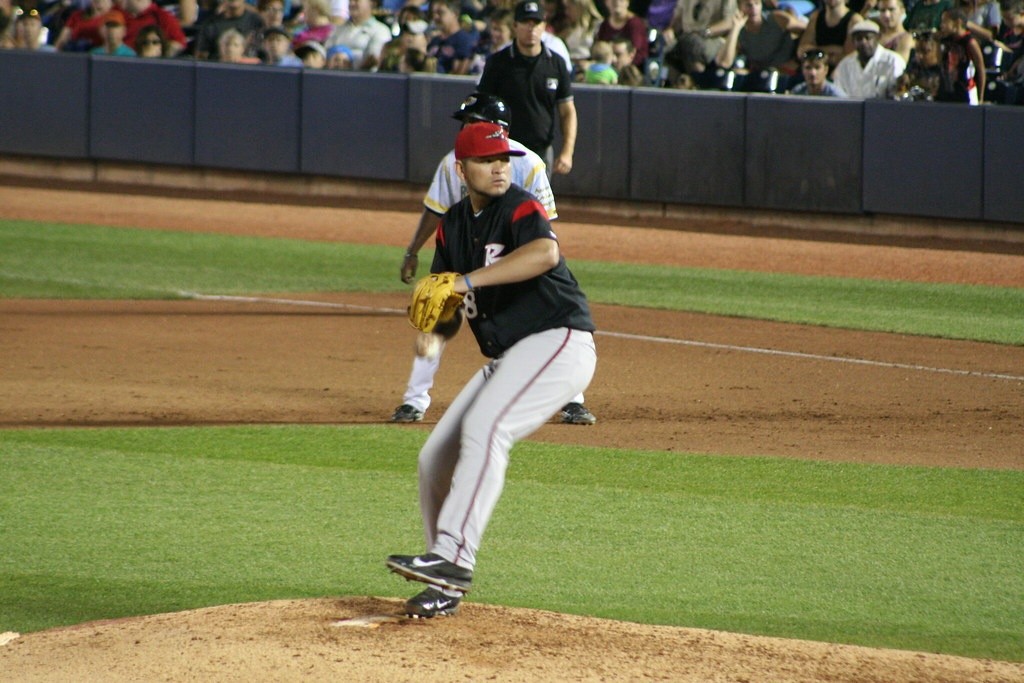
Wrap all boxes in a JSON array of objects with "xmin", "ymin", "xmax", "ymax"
[
  {"xmin": 386, "ymin": 553, "xmax": 472, "ymax": 595},
  {"xmin": 561, "ymin": 402, "xmax": 596, "ymax": 425},
  {"xmin": 392, "ymin": 405, "xmax": 423, "ymax": 423},
  {"xmin": 405, "ymin": 587, "xmax": 461, "ymax": 618}
]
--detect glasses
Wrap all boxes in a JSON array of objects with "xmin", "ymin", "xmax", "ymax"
[{"xmin": 143, "ymin": 39, "xmax": 162, "ymax": 46}]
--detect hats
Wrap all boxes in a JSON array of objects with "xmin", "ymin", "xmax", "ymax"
[
  {"xmin": 403, "ymin": 20, "xmax": 432, "ymax": 43},
  {"xmin": 326, "ymin": 45, "xmax": 352, "ymax": 63},
  {"xmin": 101, "ymin": 10, "xmax": 126, "ymax": 27},
  {"xmin": 514, "ymin": 0, "xmax": 544, "ymax": 21},
  {"xmin": 849, "ymin": 20, "xmax": 880, "ymax": 34},
  {"xmin": 293, "ymin": 40, "xmax": 326, "ymax": 62},
  {"xmin": 264, "ymin": 28, "xmax": 292, "ymax": 40},
  {"xmin": 455, "ymin": 122, "xmax": 526, "ymax": 161}
]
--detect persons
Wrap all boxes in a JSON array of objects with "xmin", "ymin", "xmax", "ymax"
[
  {"xmin": 463, "ymin": 2, "xmax": 578, "ymax": 183},
  {"xmin": 390, "ymin": 93, "xmax": 597, "ymax": 426},
  {"xmin": 383, "ymin": 121, "xmax": 599, "ymax": 621},
  {"xmin": 0, "ymin": 0, "xmax": 1024, "ymax": 112}
]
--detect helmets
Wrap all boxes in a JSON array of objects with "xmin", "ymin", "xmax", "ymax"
[{"xmin": 454, "ymin": 92, "xmax": 512, "ymax": 131}]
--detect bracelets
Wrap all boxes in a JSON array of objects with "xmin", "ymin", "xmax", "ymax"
[
  {"xmin": 404, "ymin": 252, "xmax": 418, "ymax": 257},
  {"xmin": 464, "ymin": 273, "xmax": 474, "ymax": 292}
]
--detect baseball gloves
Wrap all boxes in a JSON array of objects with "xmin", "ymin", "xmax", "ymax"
[{"xmin": 407, "ymin": 271, "xmax": 464, "ymax": 340}]
